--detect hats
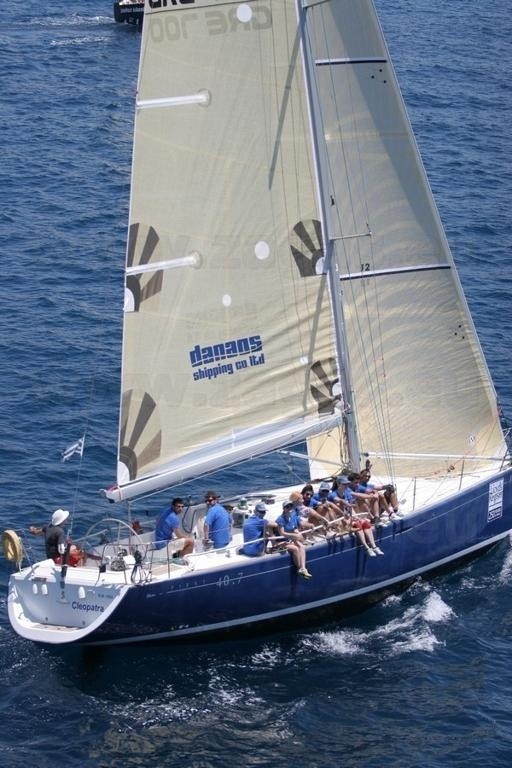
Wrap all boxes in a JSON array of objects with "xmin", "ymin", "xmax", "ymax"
[
  {"xmin": 52, "ymin": 509, "xmax": 69, "ymax": 526},
  {"xmin": 319, "ymin": 482, "xmax": 330, "ymax": 490},
  {"xmin": 282, "ymin": 501, "xmax": 293, "ymax": 509},
  {"xmin": 255, "ymin": 503, "xmax": 268, "ymax": 511},
  {"xmin": 336, "ymin": 476, "xmax": 351, "ymax": 485}
]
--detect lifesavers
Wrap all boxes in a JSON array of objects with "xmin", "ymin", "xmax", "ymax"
[{"xmin": 4, "ymin": 530, "xmax": 22, "ymax": 562}]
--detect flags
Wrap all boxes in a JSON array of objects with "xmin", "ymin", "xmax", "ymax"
[{"xmin": 61, "ymin": 435, "xmax": 86, "ymax": 463}]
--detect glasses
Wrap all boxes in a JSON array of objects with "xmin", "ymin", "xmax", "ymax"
[
  {"xmin": 205, "ymin": 499, "xmax": 214, "ymax": 502},
  {"xmin": 286, "ymin": 504, "xmax": 293, "ymax": 508},
  {"xmin": 178, "ymin": 506, "xmax": 184, "ymax": 508},
  {"xmin": 365, "ymin": 474, "xmax": 370, "ymax": 478},
  {"xmin": 322, "ymin": 490, "xmax": 329, "ymax": 492},
  {"xmin": 306, "ymin": 493, "xmax": 313, "ymax": 497}
]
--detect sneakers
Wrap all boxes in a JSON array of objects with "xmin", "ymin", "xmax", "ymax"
[
  {"xmin": 366, "ymin": 547, "xmax": 376, "ymax": 557},
  {"xmin": 300, "ymin": 574, "xmax": 309, "ymax": 581},
  {"xmin": 271, "ymin": 531, "xmax": 338, "ymax": 553},
  {"xmin": 373, "ymin": 547, "xmax": 384, "ymax": 556},
  {"xmin": 298, "ymin": 567, "xmax": 312, "ymax": 578},
  {"xmin": 375, "ymin": 511, "xmax": 406, "ymax": 527}
]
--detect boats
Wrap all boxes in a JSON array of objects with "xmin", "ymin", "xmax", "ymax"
[{"xmin": 108, "ymin": 0, "xmax": 144, "ymax": 23}]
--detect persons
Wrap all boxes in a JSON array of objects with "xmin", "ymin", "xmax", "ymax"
[
  {"xmin": 156, "ymin": 498, "xmax": 194, "ymax": 565},
  {"xmin": 244, "ymin": 470, "xmax": 404, "ymax": 580},
  {"xmin": 203, "ymin": 490, "xmax": 233, "ymax": 549},
  {"xmin": 30, "ymin": 508, "xmax": 82, "ymax": 567}
]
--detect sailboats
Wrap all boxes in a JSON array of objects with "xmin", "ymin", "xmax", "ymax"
[{"xmin": 0, "ymin": 0, "xmax": 512, "ymax": 659}]
